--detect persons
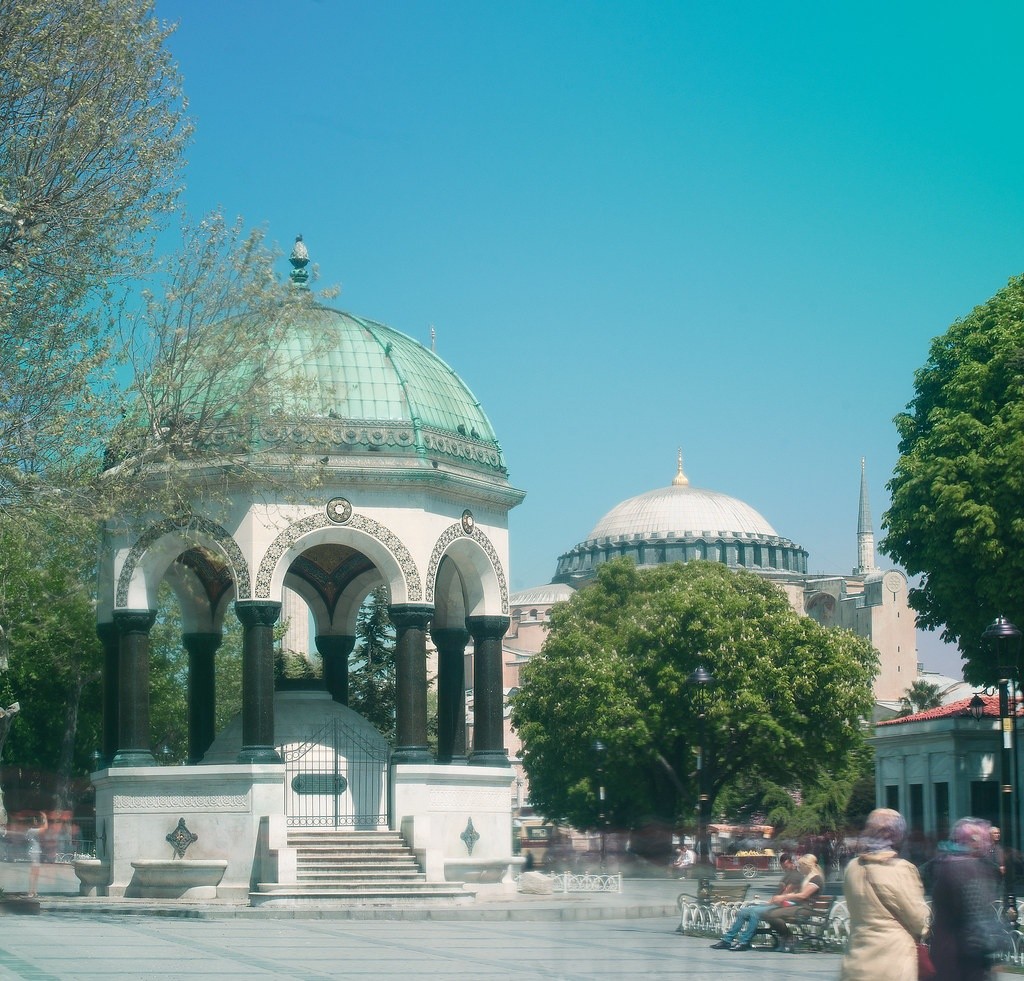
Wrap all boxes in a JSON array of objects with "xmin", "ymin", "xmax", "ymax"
[
  {"xmin": 525, "ymin": 849, "xmax": 533, "ymax": 872},
  {"xmin": 917, "ymin": 816, "xmax": 1006, "ymax": 981},
  {"xmin": 710, "ymin": 854, "xmax": 825, "ymax": 952},
  {"xmin": 25, "ymin": 810, "xmax": 48, "ymax": 899},
  {"xmin": 671, "ymin": 843, "xmax": 699, "ymax": 881},
  {"xmin": 841, "ymin": 808, "xmax": 934, "ymax": 981}
]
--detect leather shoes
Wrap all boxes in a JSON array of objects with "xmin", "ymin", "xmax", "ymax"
[
  {"xmin": 710, "ymin": 940, "xmax": 731, "ymax": 949},
  {"xmin": 729, "ymin": 944, "xmax": 747, "ymax": 951}
]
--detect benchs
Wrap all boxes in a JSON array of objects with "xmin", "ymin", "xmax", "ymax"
[
  {"xmin": 752, "ymin": 895, "xmax": 837, "ymax": 953},
  {"xmin": 675, "ymin": 884, "xmax": 751, "ymax": 931}
]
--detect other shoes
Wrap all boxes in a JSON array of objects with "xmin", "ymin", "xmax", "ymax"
[{"xmin": 774, "ymin": 944, "xmax": 795, "ymax": 952}]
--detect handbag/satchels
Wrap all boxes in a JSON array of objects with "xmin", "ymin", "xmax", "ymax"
[{"xmin": 917, "ymin": 942, "xmax": 937, "ymax": 979}]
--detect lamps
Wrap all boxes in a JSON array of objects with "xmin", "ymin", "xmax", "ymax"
[
  {"xmin": 159, "ymin": 744, "xmax": 171, "ymax": 766},
  {"xmin": 89, "ymin": 751, "xmax": 102, "ymax": 772}
]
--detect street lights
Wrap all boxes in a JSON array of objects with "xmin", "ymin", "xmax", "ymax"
[
  {"xmin": 589, "ymin": 738, "xmax": 608, "ymax": 878},
  {"xmin": 965, "ymin": 604, "xmax": 1024, "ymax": 945},
  {"xmin": 684, "ymin": 663, "xmax": 715, "ymax": 910}
]
